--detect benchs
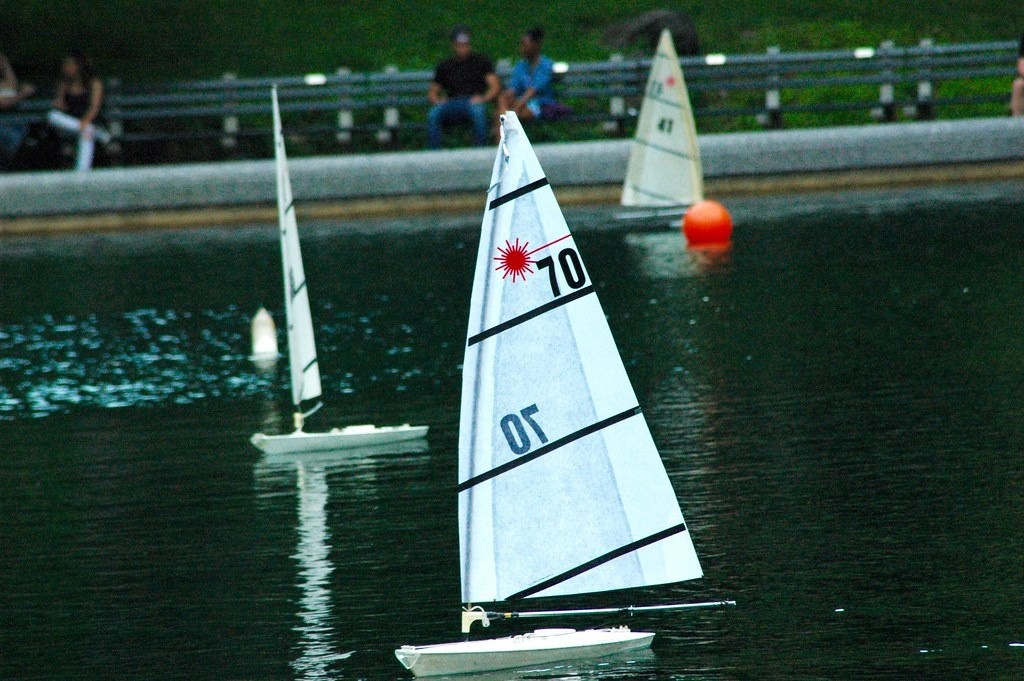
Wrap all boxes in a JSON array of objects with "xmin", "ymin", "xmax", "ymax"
[{"xmin": 0, "ymin": 35, "xmax": 1019, "ymax": 172}]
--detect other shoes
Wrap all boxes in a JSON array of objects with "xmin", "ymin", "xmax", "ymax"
[{"xmin": 99, "ymin": 132, "xmax": 119, "ymax": 154}]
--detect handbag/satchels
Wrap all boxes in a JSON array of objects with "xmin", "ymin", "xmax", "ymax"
[{"xmin": 542, "ymin": 103, "xmax": 573, "ymax": 124}]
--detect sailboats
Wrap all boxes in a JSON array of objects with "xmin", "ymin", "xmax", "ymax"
[
  {"xmin": 611, "ymin": 27, "xmax": 704, "ymax": 222},
  {"xmin": 394, "ymin": 108, "xmax": 706, "ymax": 680},
  {"xmin": 248, "ymin": 82, "xmax": 431, "ymax": 456}
]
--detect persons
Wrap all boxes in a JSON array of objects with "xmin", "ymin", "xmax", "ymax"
[
  {"xmin": 0, "ymin": 55, "xmax": 35, "ymax": 170},
  {"xmin": 1010, "ymin": 31, "xmax": 1024, "ymax": 117},
  {"xmin": 47, "ymin": 54, "xmax": 122, "ymax": 171},
  {"xmin": 495, "ymin": 28, "xmax": 557, "ymax": 146},
  {"xmin": 428, "ymin": 27, "xmax": 500, "ymax": 150}
]
